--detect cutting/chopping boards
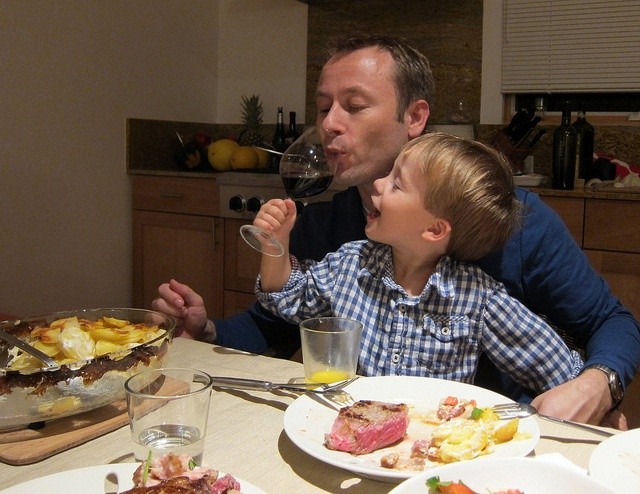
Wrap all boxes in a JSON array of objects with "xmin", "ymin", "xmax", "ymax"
[{"xmin": 1, "ymin": 374, "xmax": 191, "ymax": 466}]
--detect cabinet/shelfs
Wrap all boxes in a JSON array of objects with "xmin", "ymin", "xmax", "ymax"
[
  {"xmin": 223, "ymin": 217, "xmax": 276, "ymax": 320},
  {"xmin": 131, "ymin": 175, "xmax": 224, "ymax": 323},
  {"xmin": 583, "ymin": 199, "xmax": 640, "ymax": 430}
]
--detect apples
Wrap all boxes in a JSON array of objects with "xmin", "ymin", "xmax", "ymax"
[{"xmin": 194, "ymin": 133, "xmax": 207, "ymax": 144}]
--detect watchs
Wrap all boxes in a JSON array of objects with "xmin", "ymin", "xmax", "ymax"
[{"xmin": 582, "ymin": 364, "xmax": 625, "ymax": 408}]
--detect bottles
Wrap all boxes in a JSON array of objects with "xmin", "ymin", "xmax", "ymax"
[
  {"xmin": 285, "ymin": 111, "xmax": 300, "ymax": 149},
  {"xmin": 272, "ymin": 107, "xmax": 286, "ymax": 173},
  {"xmin": 572, "ymin": 101, "xmax": 595, "ymax": 187},
  {"xmin": 552, "ymin": 101, "xmax": 577, "ymax": 189}
]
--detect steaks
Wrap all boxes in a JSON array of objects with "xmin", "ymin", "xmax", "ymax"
[
  {"xmin": 122, "ymin": 472, "xmax": 241, "ymax": 494},
  {"xmin": 325, "ymin": 399, "xmax": 409, "ymax": 454}
]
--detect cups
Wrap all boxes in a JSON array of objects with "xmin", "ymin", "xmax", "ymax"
[
  {"xmin": 124, "ymin": 367, "xmax": 212, "ymax": 464},
  {"xmin": 299, "ymin": 317, "xmax": 361, "ymax": 386}
]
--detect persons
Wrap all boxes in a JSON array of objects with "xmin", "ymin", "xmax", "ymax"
[
  {"xmin": 145, "ymin": 29, "xmax": 638, "ymax": 428},
  {"xmin": 253, "ymin": 131, "xmax": 628, "ymax": 432}
]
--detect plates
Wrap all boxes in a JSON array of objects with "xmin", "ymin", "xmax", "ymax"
[
  {"xmin": 0, "ymin": 463, "xmax": 275, "ymax": 494},
  {"xmin": 386, "ymin": 458, "xmax": 618, "ymax": 494},
  {"xmin": 284, "ymin": 375, "xmax": 538, "ymax": 483},
  {"xmin": 590, "ymin": 428, "xmax": 640, "ymax": 494},
  {"xmin": 510, "ymin": 173, "xmax": 545, "ymax": 187}
]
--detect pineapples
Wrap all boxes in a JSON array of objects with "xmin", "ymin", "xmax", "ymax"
[{"xmin": 237, "ymin": 93, "xmax": 265, "ymax": 147}]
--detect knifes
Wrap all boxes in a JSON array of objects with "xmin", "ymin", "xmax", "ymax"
[
  {"xmin": 503, "ymin": 109, "xmax": 546, "ymax": 147},
  {"xmin": 193, "ymin": 373, "xmax": 345, "ymax": 399}
]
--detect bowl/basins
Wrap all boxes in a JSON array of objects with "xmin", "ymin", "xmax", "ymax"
[{"xmin": 0, "ymin": 308, "xmax": 177, "ymax": 430}]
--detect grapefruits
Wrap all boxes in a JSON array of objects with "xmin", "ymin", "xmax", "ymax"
[{"xmin": 207, "ymin": 138, "xmax": 240, "ymax": 170}]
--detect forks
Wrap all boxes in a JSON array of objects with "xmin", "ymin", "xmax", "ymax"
[{"xmin": 492, "ymin": 403, "xmax": 614, "ymax": 438}]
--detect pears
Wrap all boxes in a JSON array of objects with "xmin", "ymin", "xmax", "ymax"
[
  {"xmin": 251, "ymin": 146, "xmax": 272, "ymax": 169},
  {"xmin": 230, "ymin": 145, "xmax": 257, "ymax": 169}
]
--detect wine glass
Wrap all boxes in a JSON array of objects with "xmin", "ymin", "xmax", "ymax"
[{"xmin": 239, "ymin": 126, "xmax": 339, "ymax": 257}]
